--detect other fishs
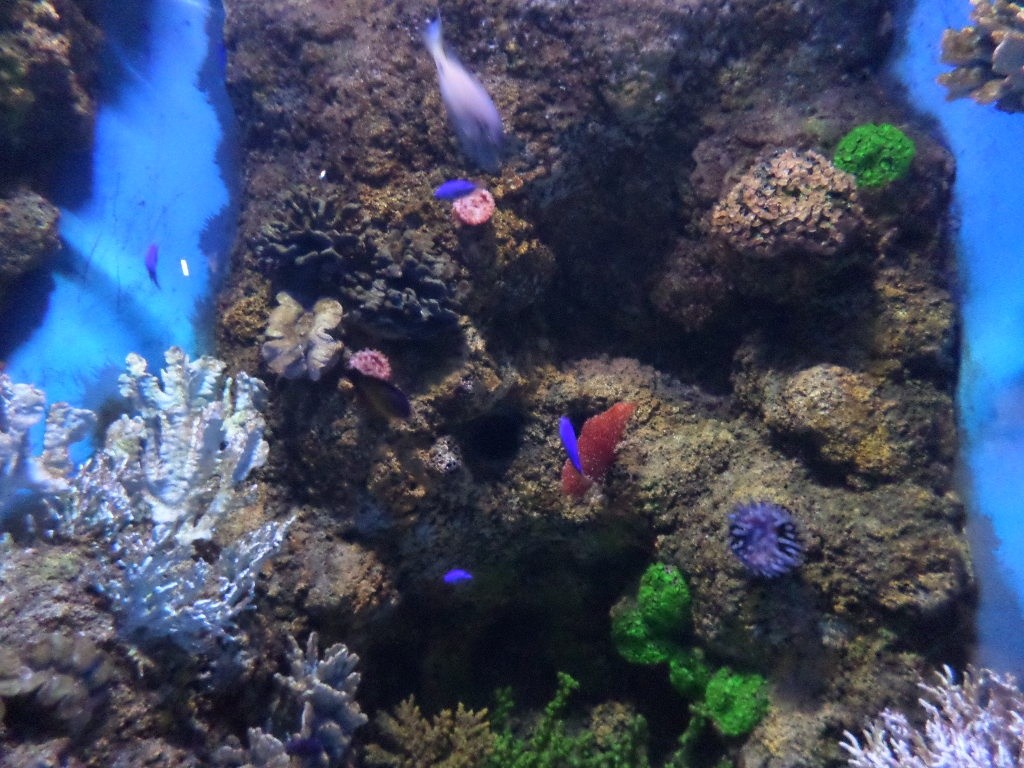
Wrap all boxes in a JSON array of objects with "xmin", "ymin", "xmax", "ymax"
[
  {"xmin": 419, "ymin": 4, "xmax": 504, "ymax": 201},
  {"xmin": 144, "ymin": 244, "xmax": 581, "ymax": 586}
]
